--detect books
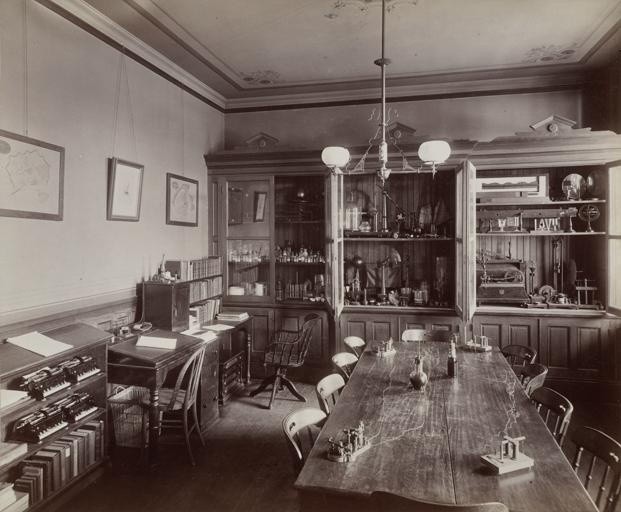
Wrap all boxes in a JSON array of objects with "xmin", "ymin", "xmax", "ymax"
[
  {"xmin": 168, "ymin": 256, "xmax": 221, "ymax": 328},
  {"xmin": 0, "ymin": 419, "xmax": 106, "ymax": 511}
]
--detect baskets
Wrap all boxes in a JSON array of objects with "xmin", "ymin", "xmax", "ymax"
[{"xmin": 107, "ymin": 384, "xmax": 152, "ymax": 448}]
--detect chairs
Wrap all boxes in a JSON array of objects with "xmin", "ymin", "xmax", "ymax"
[
  {"xmin": 140, "ymin": 346, "xmax": 206, "ymax": 466},
  {"xmin": 249, "ymin": 313, "xmax": 321, "ymax": 410}
]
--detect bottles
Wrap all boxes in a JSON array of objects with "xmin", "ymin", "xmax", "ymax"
[
  {"xmin": 277, "ymin": 244, "xmax": 322, "ymax": 263},
  {"xmin": 448, "ymin": 340, "xmax": 458, "ymax": 377}
]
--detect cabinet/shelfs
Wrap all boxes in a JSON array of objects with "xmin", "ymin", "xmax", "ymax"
[
  {"xmin": 0, "ymin": 322, "xmax": 112, "ymax": 512},
  {"xmin": 218, "ymin": 164, "xmax": 334, "ymax": 384},
  {"xmin": 334, "ymin": 163, "xmax": 467, "ymax": 360},
  {"xmin": 161, "ymin": 274, "xmax": 254, "ymax": 435},
  {"xmin": 467, "ymin": 162, "xmax": 621, "ymax": 409},
  {"xmin": 136, "ymin": 280, "xmax": 190, "ymax": 333}
]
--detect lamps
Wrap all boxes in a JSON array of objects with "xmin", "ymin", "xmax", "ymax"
[{"xmin": 321, "ymin": 0, "xmax": 452, "ymax": 189}]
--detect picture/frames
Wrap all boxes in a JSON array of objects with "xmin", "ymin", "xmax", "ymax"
[
  {"xmin": 0, "ymin": 129, "xmax": 65, "ymax": 221},
  {"xmin": 107, "ymin": 157, "xmax": 144, "ymax": 223},
  {"xmin": 166, "ymin": 172, "xmax": 199, "ymax": 226}
]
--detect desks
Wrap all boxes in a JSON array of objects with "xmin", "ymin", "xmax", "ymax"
[{"xmin": 104, "ymin": 321, "xmax": 204, "ymax": 470}]
